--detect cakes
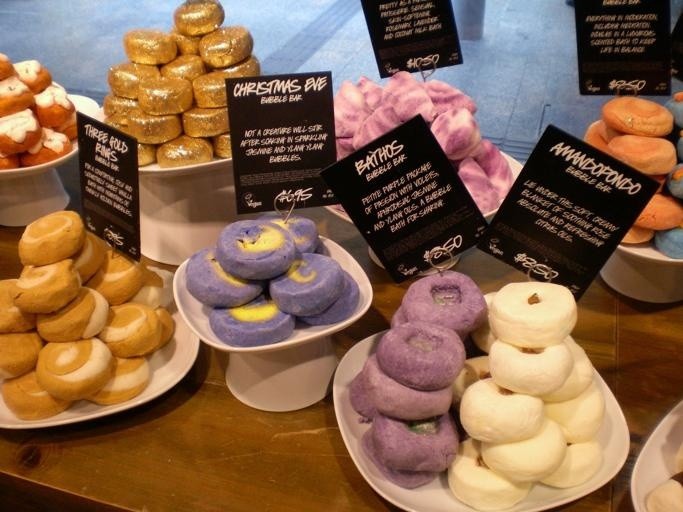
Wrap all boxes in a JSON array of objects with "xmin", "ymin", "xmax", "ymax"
[
  {"xmin": 0, "ymin": 52, "xmax": 80, "ymax": 169},
  {"xmin": 0, "ymin": 210, "xmax": 175, "ymax": 422},
  {"xmin": 185, "ymin": 211, "xmax": 360, "ymax": 349},
  {"xmin": 103, "ymin": 1, "xmax": 261, "ymax": 172},
  {"xmin": 332, "ymin": 71, "xmax": 514, "ymax": 215}
]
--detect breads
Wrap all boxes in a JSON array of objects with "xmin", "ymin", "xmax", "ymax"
[
  {"xmin": 644, "ymin": 470, "xmax": 683, "ymax": 512},
  {"xmin": 349, "ymin": 269, "xmax": 606, "ymax": 511},
  {"xmin": 583, "ymin": 90, "xmax": 683, "ymax": 258}
]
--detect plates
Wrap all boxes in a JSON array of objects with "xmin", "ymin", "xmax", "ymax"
[
  {"xmin": 333, "ymin": 328, "xmax": 632, "ymax": 511},
  {"xmin": 632, "ymin": 399, "xmax": 683, "ymax": 512},
  {"xmin": 0, "ymin": 266, "xmax": 201, "ymax": 429}
]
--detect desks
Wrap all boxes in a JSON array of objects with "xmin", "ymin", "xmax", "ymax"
[{"xmin": 0, "ymin": 225, "xmax": 679, "ymax": 511}]
